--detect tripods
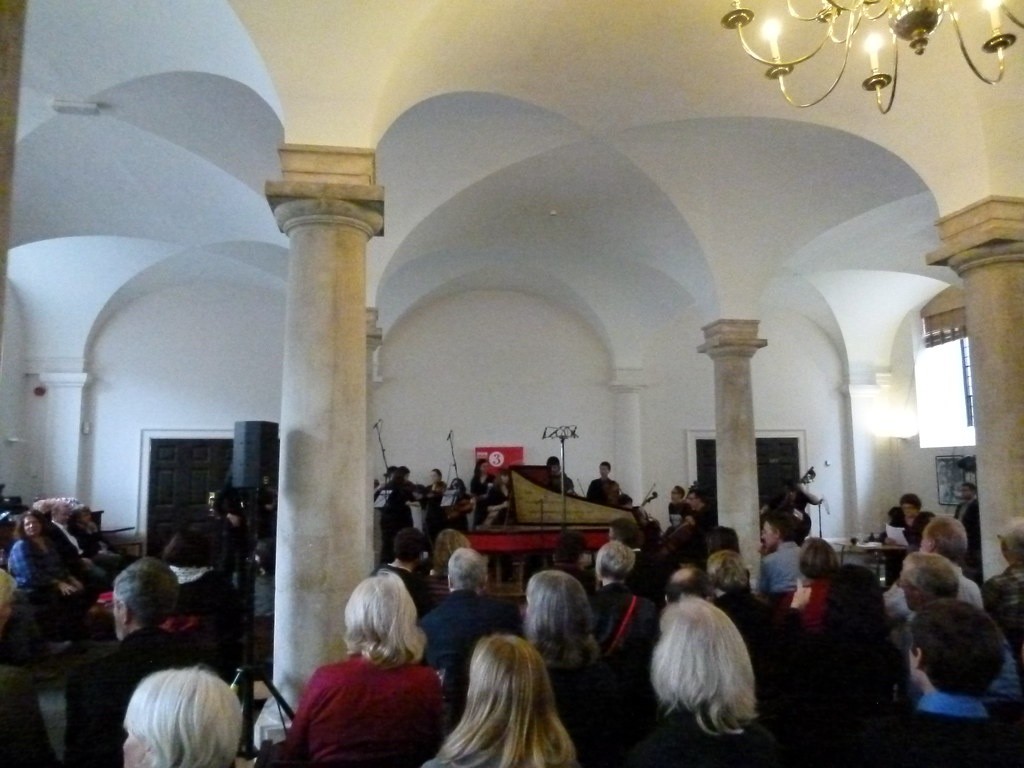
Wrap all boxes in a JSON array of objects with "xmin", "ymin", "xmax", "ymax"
[{"xmin": 230, "ymin": 488, "xmax": 296, "ymax": 761}]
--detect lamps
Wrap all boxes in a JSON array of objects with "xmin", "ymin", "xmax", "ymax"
[{"xmin": 721, "ymin": 0, "xmax": 1024, "ymax": 115}]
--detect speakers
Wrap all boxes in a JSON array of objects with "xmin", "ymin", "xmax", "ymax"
[{"xmin": 232, "ymin": 421, "xmax": 279, "ymax": 488}]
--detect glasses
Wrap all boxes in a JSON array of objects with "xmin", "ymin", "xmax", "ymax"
[{"xmin": 896, "ymin": 580, "xmax": 919, "ymax": 589}]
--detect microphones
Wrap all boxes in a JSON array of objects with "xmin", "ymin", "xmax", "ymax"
[
  {"xmin": 373, "ymin": 419, "xmax": 381, "ymax": 428},
  {"xmin": 447, "ymin": 431, "xmax": 452, "ymax": 440},
  {"xmin": 542, "ymin": 426, "xmax": 579, "ymax": 439}
]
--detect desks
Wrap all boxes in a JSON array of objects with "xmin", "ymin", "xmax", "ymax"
[{"xmin": 835, "ymin": 541, "xmax": 908, "ymax": 594}]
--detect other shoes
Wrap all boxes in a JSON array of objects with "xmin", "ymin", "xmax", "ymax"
[{"xmin": 48, "ymin": 640, "xmax": 71, "ymax": 653}]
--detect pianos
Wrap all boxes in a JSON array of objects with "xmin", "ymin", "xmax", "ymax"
[{"xmin": 464, "ymin": 465, "xmax": 644, "ymax": 587}]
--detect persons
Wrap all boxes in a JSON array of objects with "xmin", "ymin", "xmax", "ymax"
[{"xmin": 0, "ymin": 456, "xmax": 1024, "ymax": 768}]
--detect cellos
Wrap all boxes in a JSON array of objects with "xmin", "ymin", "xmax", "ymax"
[{"xmin": 640, "ymin": 490, "xmax": 659, "ymax": 505}]
